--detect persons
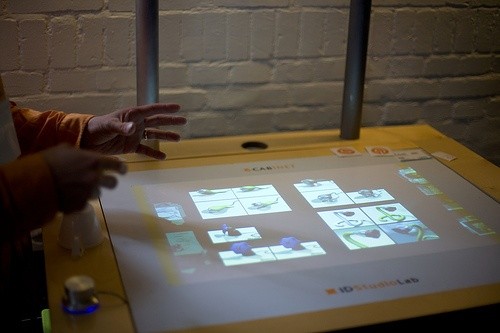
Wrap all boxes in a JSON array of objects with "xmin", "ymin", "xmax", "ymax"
[{"xmin": 0, "ymin": 72, "xmax": 187, "ymax": 270}]
